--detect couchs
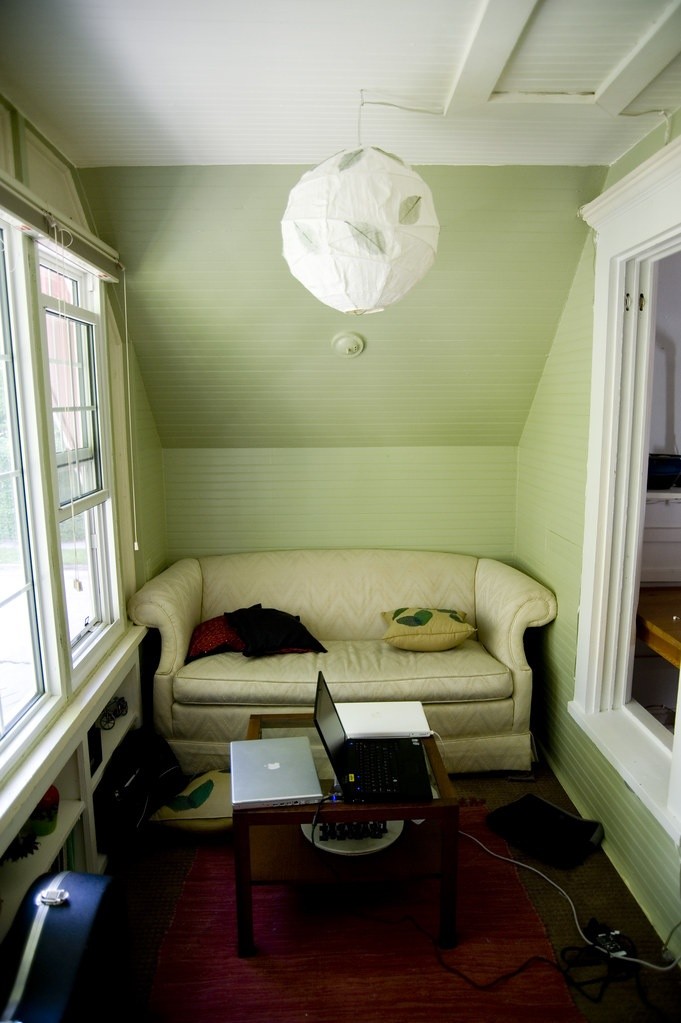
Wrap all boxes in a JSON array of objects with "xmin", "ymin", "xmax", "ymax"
[{"xmin": 126, "ymin": 549, "xmax": 557, "ymax": 778}]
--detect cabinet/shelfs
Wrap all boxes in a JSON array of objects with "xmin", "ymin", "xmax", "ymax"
[{"xmin": 0, "ymin": 627, "xmax": 151, "ymax": 940}]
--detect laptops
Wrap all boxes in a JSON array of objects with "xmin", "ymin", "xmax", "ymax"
[
  {"xmin": 335, "ymin": 699, "xmax": 430, "ymax": 740},
  {"xmin": 311, "ymin": 670, "xmax": 432, "ymax": 805},
  {"xmin": 228, "ymin": 737, "xmax": 323, "ymax": 809}
]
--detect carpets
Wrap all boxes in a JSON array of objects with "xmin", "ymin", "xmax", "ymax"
[{"xmin": 142, "ymin": 799, "xmax": 586, "ymax": 1023}]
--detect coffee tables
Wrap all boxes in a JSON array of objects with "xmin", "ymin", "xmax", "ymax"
[{"xmin": 232, "ymin": 713, "xmax": 464, "ymax": 958}]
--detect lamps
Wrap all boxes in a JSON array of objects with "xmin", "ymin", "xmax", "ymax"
[{"xmin": 280, "ymin": 144, "xmax": 442, "ymax": 316}]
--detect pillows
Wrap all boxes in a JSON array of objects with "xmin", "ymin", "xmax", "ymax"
[
  {"xmin": 184, "ymin": 603, "xmax": 328, "ymax": 665},
  {"xmin": 149, "ymin": 769, "xmax": 233, "ymax": 831},
  {"xmin": 380, "ymin": 607, "xmax": 478, "ymax": 651}
]
